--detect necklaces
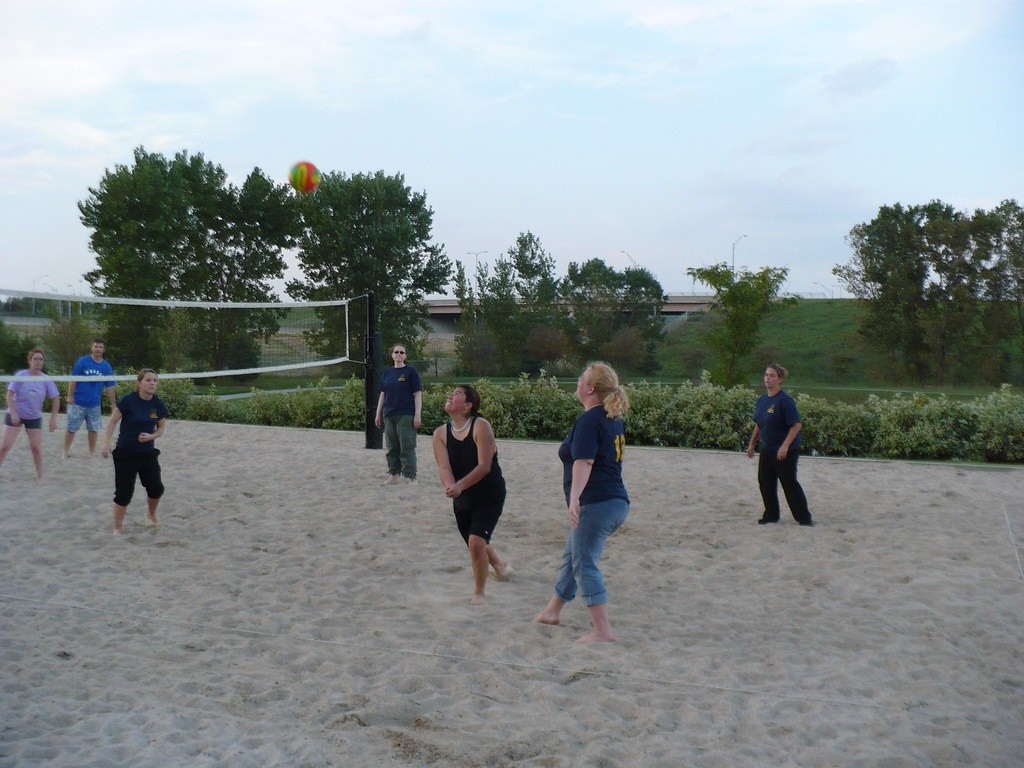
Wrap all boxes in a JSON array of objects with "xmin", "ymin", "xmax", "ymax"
[{"xmin": 452, "ymin": 416, "xmax": 472, "ymax": 432}]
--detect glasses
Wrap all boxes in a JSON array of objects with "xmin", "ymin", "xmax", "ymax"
[{"xmin": 393, "ymin": 350, "xmax": 406, "ymax": 355}]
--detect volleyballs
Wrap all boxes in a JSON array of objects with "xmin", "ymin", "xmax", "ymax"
[{"xmin": 289, "ymin": 160, "xmax": 321, "ymax": 193}]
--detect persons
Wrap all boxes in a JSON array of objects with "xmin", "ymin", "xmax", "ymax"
[
  {"xmin": 64, "ymin": 338, "xmax": 116, "ymax": 460},
  {"xmin": 0, "ymin": 349, "xmax": 61, "ymax": 487},
  {"xmin": 432, "ymin": 385, "xmax": 513, "ymax": 606},
  {"xmin": 101, "ymin": 367, "xmax": 169, "ymax": 536},
  {"xmin": 747, "ymin": 364, "xmax": 813, "ymax": 526},
  {"xmin": 533, "ymin": 361, "xmax": 630, "ymax": 645},
  {"xmin": 375, "ymin": 343, "xmax": 422, "ymax": 488}
]
